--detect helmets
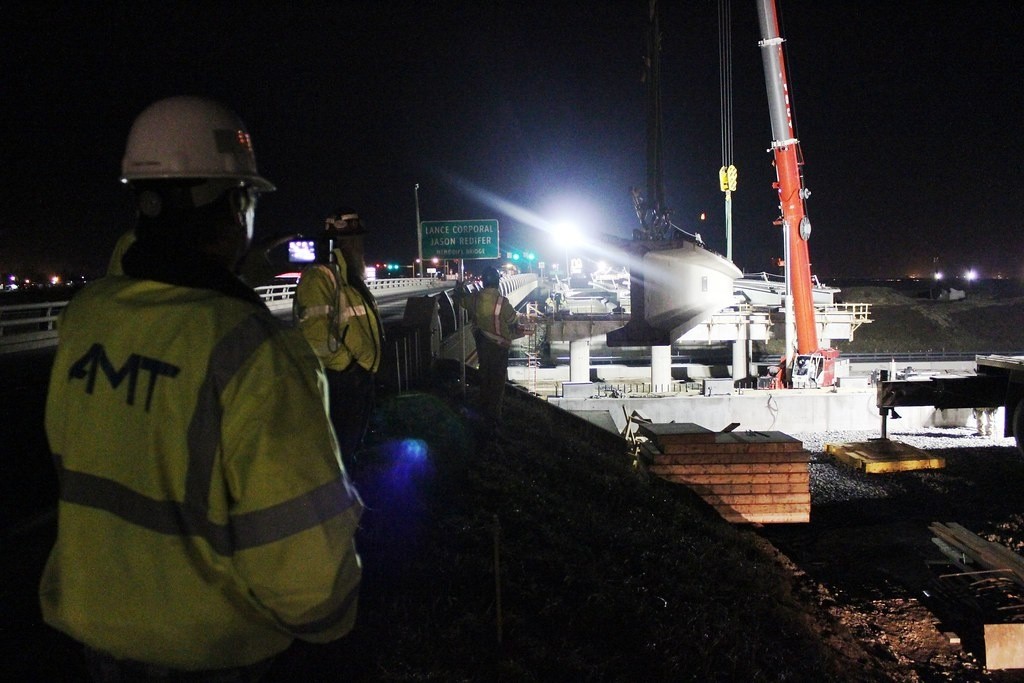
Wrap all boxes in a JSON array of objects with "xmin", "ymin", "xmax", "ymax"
[
  {"xmin": 482, "ymin": 266, "xmax": 498, "ymax": 284},
  {"xmin": 314, "ymin": 205, "xmax": 370, "ymax": 238},
  {"xmin": 120, "ymin": 95, "xmax": 276, "ymax": 193}
]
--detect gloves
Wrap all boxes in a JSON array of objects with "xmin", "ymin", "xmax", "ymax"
[{"xmin": 343, "ymin": 365, "xmax": 373, "ymax": 401}]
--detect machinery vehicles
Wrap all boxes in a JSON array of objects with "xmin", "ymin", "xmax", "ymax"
[{"xmin": 717, "ymin": 0, "xmax": 850, "ymax": 391}]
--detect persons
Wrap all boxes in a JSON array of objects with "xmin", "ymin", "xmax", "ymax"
[
  {"xmin": 107, "ymin": 227, "xmax": 292, "ymax": 284},
  {"xmin": 804, "ymin": 359, "xmax": 814, "ymax": 378},
  {"xmin": 451, "ymin": 267, "xmax": 523, "ymax": 420},
  {"xmin": 292, "ymin": 206, "xmax": 387, "ymax": 452},
  {"xmin": 38, "ymin": 96, "xmax": 367, "ymax": 683}
]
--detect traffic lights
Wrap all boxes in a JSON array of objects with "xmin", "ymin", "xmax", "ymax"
[
  {"xmin": 387, "ymin": 263, "xmax": 399, "ymax": 270},
  {"xmin": 513, "ymin": 253, "xmax": 520, "ymax": 260},
  {"xmin": 528, "ymin": 254, "xmax": 535, "ymax": 260},
  {"xmin": 376, "ymin": 263, "xmax": 386, "ymax": 268}
]
